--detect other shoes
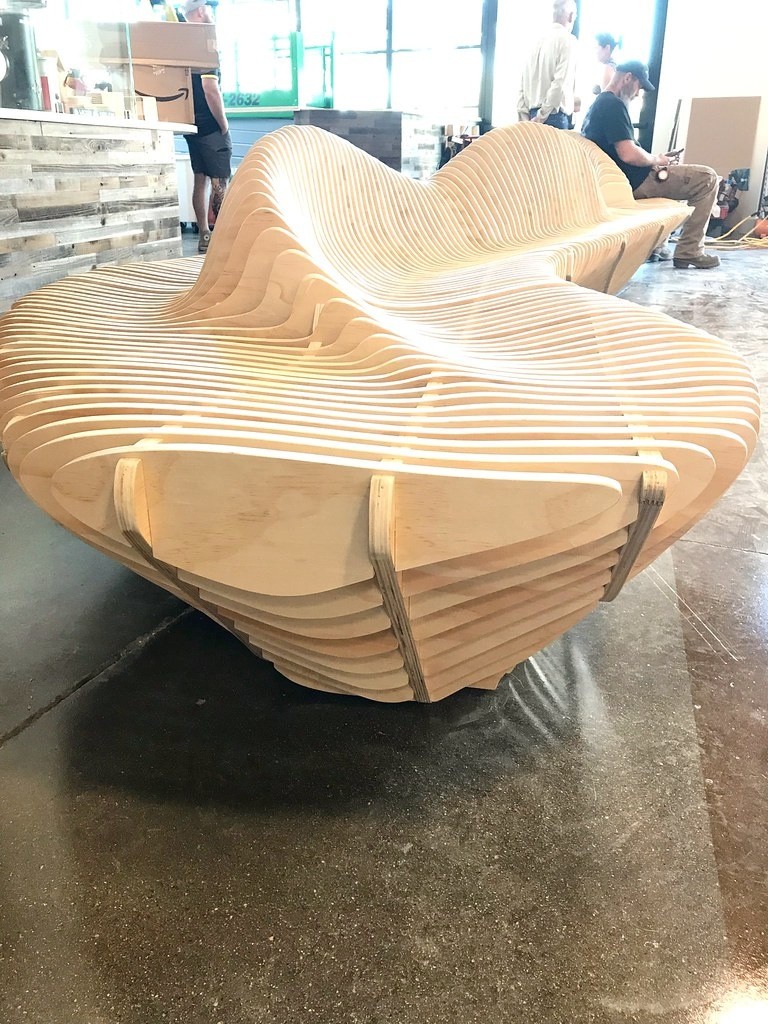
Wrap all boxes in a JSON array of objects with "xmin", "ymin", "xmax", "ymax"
[{"xmin": 198, "ymin": 240, "xmax": 210, "ymax": 252}]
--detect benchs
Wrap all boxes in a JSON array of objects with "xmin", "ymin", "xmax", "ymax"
[{"xmin": 0, "ymin": 122, "xmax": 760, "ymax": 704}]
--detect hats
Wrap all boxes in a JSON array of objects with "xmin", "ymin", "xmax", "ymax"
[
  {"xmin": 615, "ymin": 62, "xmax": 655, "ymax": 92},
  {"xmin": 181, "ymin": 1, "xmax": 220, "ymax": 11}
]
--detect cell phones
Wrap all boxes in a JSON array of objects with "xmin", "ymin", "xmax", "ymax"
[{"xmin": 668, "ymin": 149, "xmax": 685, "ymax": 158}]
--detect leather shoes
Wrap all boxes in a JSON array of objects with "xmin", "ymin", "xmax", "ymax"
[
  {"xmin": 649, "ymin": 246, "xmax": 674, "ymax": 261},
  {"xmin": 672, "ymin": 253, "xmax": 720, "ymax": 268}
]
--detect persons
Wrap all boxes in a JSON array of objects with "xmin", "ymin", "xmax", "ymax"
[
  {"xmin": 592, "ymin": 33, "xmax": 620, "ymax": 95},
  {"xmin": 517, "ymin": 0, "xmax": 579, "ymax": 128},
  {"xmin": 581, "ymin": 60, "xmax": 721, "ymax": 270},
  {"xmin": 182, "ymin": 1, "xmax": 232, "ymax": 255}
]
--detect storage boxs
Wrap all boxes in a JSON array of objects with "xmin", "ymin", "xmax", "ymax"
[
  {"xmin": 71, "ymin": 22, "xmax": 219, "ymax": 124},
  {"xmin": 684, "ymin": 97, "xmax": 768, "ymax": 238}
]
